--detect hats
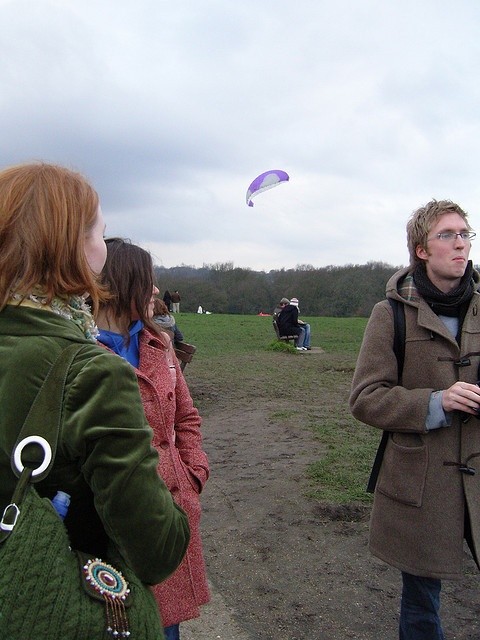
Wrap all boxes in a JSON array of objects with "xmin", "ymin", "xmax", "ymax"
[{"xmin": 290, "ymin": 298, "xmax": 300, "ymax": 313}]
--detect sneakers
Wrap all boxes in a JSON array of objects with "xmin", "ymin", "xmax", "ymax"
[{"xmin": 296, "ymin": 347, "xmax": 307, "ymax": 351}]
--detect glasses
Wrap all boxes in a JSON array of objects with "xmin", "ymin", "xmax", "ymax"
[{"xmin": 426, "ymin": 231, "xmax": 476, "ymax": 242}]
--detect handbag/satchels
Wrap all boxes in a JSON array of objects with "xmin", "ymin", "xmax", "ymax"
[{"xmin": 0, "ymin": 343, "xmax": 165, "ymax": 640}]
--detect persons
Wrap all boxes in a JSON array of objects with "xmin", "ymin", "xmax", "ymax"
[
  {"xmin": 162, "ymin": 290, "xmax": 172, "ymax": 311},
  {"xmin": 1, "ymin": 162, "xmax": 190, "ymax": 587},
  {"xmin": 279, "ymin": 297, "xmax": 307, "ymax": 351},
  {"xmin": 153, "ymin": 298, "xmax": 183, "ymax": 343},
  {"xmin": 348, "ymin": 199, "xmax": 480, "ymax": 640},
  {"xmin": 272, "ymin": 298, "xmax": 310, "ymax": 349},
  {"xmin": 171, "ymin": 290, "xmax": 181, "ymax": 312},
  {"xmin": 85, "ymin": 237, "xmax": 210, "ymax": 639}
]
